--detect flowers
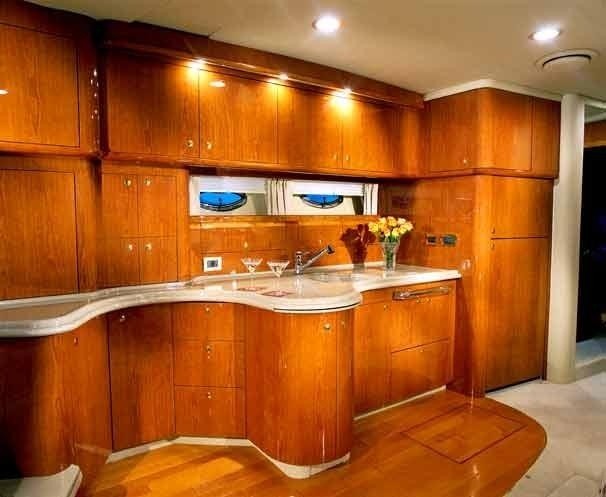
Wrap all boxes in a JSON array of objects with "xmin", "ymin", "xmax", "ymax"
[{"xmin": 367, "ymin": 216, "xmax": 414, "ymax": 269}]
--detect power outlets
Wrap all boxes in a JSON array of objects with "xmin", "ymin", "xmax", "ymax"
[{"xmin": 202, "ymin": 256, "xmax": 222, "ymax": 273}]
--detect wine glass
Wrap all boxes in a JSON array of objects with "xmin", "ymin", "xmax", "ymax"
[
  {"xmin": 241, "ymin": 258, "xmax": 263, "ymax": 291},
  {"xmin": 266, "ymin": 260, "xmax": 290, "ymax": 297}
]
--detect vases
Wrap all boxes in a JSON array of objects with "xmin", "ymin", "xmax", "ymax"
[{"xmin": 380, "ymin": 239, "xmax": 399, "ymax": 270}]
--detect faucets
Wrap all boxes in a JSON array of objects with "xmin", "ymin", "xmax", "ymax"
[{"xmin": 294, "ymin": 245, "xmax": 335, "ymax": 274}]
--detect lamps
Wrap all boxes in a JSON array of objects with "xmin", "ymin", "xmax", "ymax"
[{"xmin": 534, "ymin": 48, "xmax": 598, "ymax": 74}]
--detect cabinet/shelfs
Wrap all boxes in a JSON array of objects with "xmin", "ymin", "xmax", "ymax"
[
  {"xmin": 1, "ymin": 158, "xmax": 99, "ymax": 301},
  {"xmin": 390, "ymin": 281, "xmax": 457, "ymax": 405},
  {"xmin": 309, "ymin": 92, "xmax": 396, "ymax": 180},
  {"xmin": 478, "ymin": 88, "xmax": 559, "ymax": 178},
  {"xmin": 94, "ymin": 163, "xmax": 191, "ymax": 287},
  {"xmin": 278, "ymin": 85, "xmax": 309, "ymax": 176},
  {"xmin": 103, "ymin": 45, "xmax": 278, "ymax": 174},
  {"xmin": 353, "ymin": 289, "xmax": 390, "ymax": 420},
  {"xmin": 247, "ymin": 306, "xmax": 354, "ymax": 479},
  {"xmin": 421, "ymin": 87, "xmax": 478, "ymax": 178},
  {"xmin": 174, "ymin": 303, "xmax": 248, "ymax": 445},
  {"xmin": 380, "ymin": 178, "xmax": 554, "ymax": 398},
  {"xmin": 0, "ymin": 23, "xmax": 101, "ymax": 158},
  {"xmin": 190, "ymin": 216, "xmax": 298, "ymax": 277},
  {"xmin": 0, "ymin": 313, "xmax": 112, "ymax": 490},
  {"xmin": 299, "ymin": 215, "xmax": 385, "ymax": 267},
  {"xmin": 395, "ymin": 108, "xmax": 421, "ymax": 180},
  {"xmin": 107, "ymin": 303, "xmax": 174, "ymax": 464}
]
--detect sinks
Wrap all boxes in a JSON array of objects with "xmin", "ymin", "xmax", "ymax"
[{"xmin": 310, "ymin": 274, "xmax": 371, "ymax": 283}]
